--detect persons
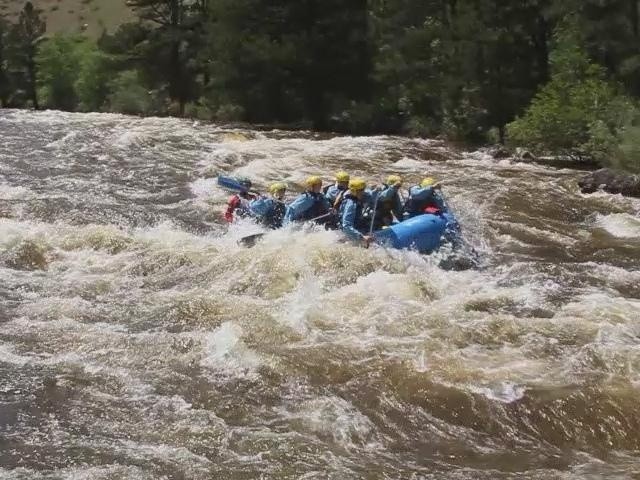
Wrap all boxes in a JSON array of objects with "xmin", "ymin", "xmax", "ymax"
[{"xmin": 226, "ymin": 172, "xmax": 444, "ymax": 249}]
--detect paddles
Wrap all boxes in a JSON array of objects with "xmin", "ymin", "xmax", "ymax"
[
  {"xmin": 218, "ymin": 176, "xmax": 261, "ymax": 195},
  {"xmin": 239, "ymin": 212, "xmax": 331, "ymax": 243}
]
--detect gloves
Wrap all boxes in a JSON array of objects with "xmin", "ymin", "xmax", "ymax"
[{"xmin": 433, "ymin": 182, "xmax": 442, "ymax": 189}]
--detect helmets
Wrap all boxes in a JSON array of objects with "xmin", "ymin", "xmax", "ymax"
[
  {"xmin": 269, "ymin": 183, "xmax": 286, "ymax": 195},
  {"xmin": 336, "ymin": 171, "xmax": 366, "ymax": 190},
  {"xmin": 239, "ymin": 178, "xmax": 252, "ymax": 188},
  {"xmin": 305, "ymin": 176, "xmax": 322, "ymax": 189},
  {"xmin": 421, "ymin": 178, "xmax": 433, "ymax": 187},
  {"xmin": 387, "ymin": 175, "xmax": 400, "ymax": 185}
]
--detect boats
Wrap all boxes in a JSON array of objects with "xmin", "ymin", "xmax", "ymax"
[{"xmin": 368, "ymin": 211, "xmax": 459, "ymax": 255}]
